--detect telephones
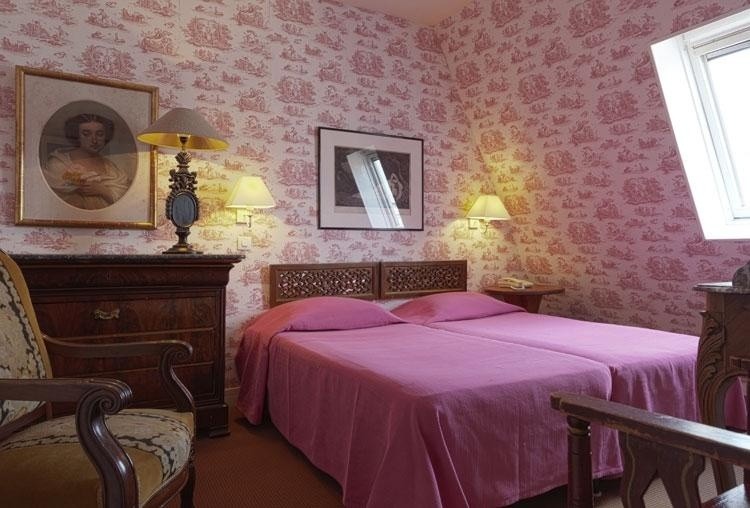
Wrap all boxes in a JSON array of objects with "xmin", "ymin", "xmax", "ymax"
[{"xmin": 497, "ymin": 277, "xmax": 533, "ymax": 291}]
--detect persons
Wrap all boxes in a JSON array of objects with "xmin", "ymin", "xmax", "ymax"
[{"xmin": 39, "ymin": 111, "xmax": 133, "ymax": 210}]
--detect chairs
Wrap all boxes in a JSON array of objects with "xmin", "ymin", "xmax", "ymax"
[{"xmin": 1, "ymin": 249, "xmax": 197, "ymax": 507}]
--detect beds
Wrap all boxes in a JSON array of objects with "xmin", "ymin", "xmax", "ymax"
[{"xmin": 244, "ymin": 257, "xmax": 744, "ymax": 508}]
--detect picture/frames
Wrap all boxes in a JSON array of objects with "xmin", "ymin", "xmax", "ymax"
[
  {"xmin": 12, "ymin": 62, "xmax": 161, "ymax": 232},
  {"xmin": 317, "ymin": 125, "xmax": 425, "ymax": 231}
]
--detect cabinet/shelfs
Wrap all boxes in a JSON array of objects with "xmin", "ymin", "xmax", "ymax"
[{"xmin": 0, "ymin": 249, "xmax": 247, "ymax": 442}]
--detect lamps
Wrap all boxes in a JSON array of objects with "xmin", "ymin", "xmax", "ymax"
[
  {"xmin": 135, "ymin": 106, "xmax": 277, "ymax": 256},
  {"xmin": 464, "ymin": 194, "xmax": 513, "ymax": 237}
]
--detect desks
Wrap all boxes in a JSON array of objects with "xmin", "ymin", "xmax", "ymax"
[{"xmin": 484, "ymin": 284, "xmax": 563, "ymax": 313}]
